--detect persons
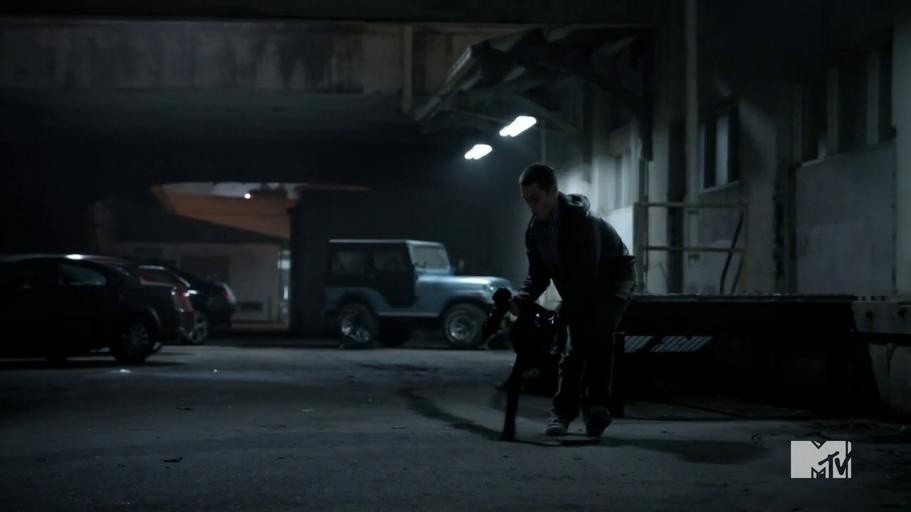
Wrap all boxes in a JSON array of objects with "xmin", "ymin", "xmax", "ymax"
[{"xmin": 504, "ymin": 162, "xmax": 638, "ymax": 437}]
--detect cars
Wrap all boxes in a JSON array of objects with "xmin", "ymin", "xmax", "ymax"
[{"xmin": 0, "ymin": 247, "xmax": 243, "ymax": 371}]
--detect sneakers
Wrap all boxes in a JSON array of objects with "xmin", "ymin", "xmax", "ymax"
[
  {"xmin": 584, "ymin": 408, "xmax": 611, "ymax": 437},
  {"xmin": 545, "ymin": 413, "xmax": 566, "ymax": 434}
]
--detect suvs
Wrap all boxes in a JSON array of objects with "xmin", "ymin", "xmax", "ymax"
[{"xmin": 315, "ymin": 235, "xmax": 514, "ymax": 351}]
